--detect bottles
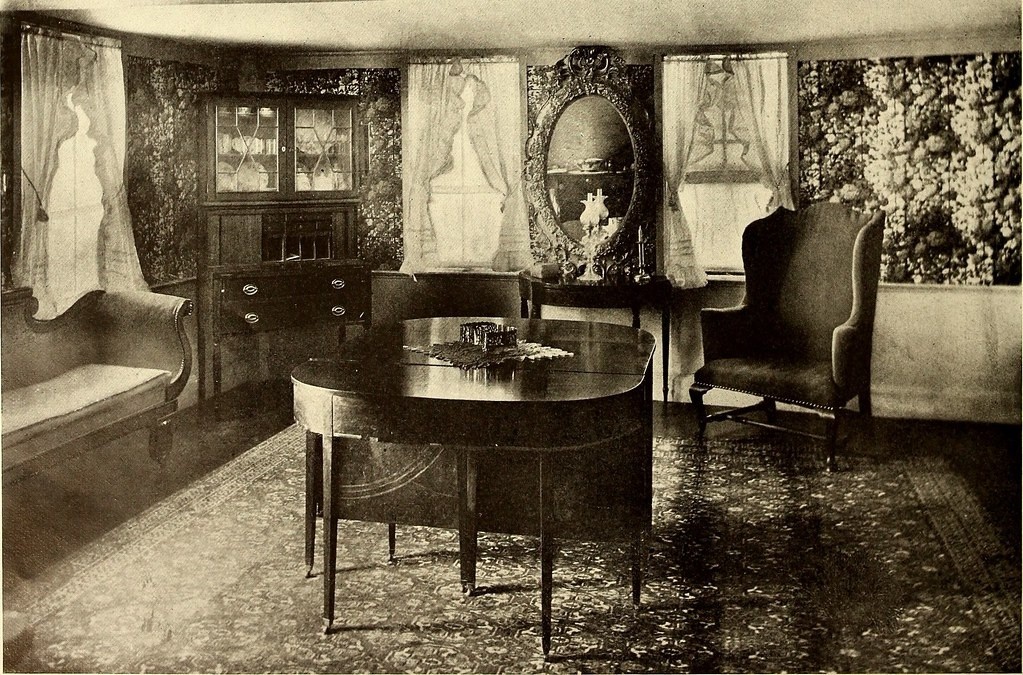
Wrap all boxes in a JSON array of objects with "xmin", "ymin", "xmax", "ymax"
[
  {"xmin": 330, "ymin": 163, "xmax": 345, "ymax": 190},
  {"xmin": 296, "ymin": 166, "xmax": 311, "ymax": 191}
]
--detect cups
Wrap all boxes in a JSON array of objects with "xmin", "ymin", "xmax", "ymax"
[
  {"xmin": 266, "ymin": 138, "xmax": 276, "ymax": 155},
  {"xmin": 238, "ymin": 107, "xmax": 251, "ymax": 113},
  {"xmin": 261, "ymin": 108, "xmax": 271, "ymax": 112},
  {"xmin": 219, "ymin": 107, "xmax": 228, "ymax": 112}
]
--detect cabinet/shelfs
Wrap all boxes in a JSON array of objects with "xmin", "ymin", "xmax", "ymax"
[{"xmin": 193, "ymin": 91, "xmax": 374, "ymax": 422}]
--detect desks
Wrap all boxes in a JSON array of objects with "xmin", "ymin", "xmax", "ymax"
[
  {"xmin": 289, "ymin": 316, "xmax": 657, "ymax": 655},
  {"xmin": 519, "ymin": 271, "xmax": 671, "ymax": 418}
]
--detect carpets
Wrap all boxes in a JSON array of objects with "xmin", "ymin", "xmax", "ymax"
[{"xmin": 3, "ymin": 437, "xmax": 1022, "ymax": 675}]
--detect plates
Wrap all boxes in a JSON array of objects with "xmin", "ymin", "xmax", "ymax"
[
  {"xmin": 219, "ymin": 112, "xmax": 230, "ymax": 114},
  {"xmin": 238, "ymin": 113, "xmax": 252, "ymax": 116},
  {"xmin": 260, "ymin": 112, "xmax": 274, "ymax": 117},
  {"xmin": 296, "ymin": 110, "xmax": 337, "ymax": 154},
  {"xmin": 256, "ymin": 139, "xmax": 264, "ymax": 154},
  {"xmin": 217, "ymin": 162, "xmax": 268, "ymax": 191}
]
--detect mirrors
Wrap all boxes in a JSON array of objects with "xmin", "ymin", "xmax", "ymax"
[{"xmin": 525, "ymin": 46, "xmax": 650, "ymax": 275}]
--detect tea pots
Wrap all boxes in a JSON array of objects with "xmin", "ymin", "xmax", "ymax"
[
  {"xmin": 233, "ymin": 136, "xmax": 256, "ymax": 153},
  {"xmin": 313, "ymin": 171, "xmax": 332, "ymax": 191}
]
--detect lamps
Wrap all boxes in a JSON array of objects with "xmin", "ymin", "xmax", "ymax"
[
  {"xmin": 592, "ymin": 189, "xmax": 609, "ymax": 244},
  {"xmin": 576, "ymin": 192, "xmax": 602, "ymax": 280}
]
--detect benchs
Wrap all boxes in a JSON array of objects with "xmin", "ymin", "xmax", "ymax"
[{"xmin": 1, "ymin": 287, "xmax": 195, "ymax": 488}]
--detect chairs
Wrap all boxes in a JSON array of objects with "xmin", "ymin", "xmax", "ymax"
[{"xmin": 689, "ymin": 203, "xmax": 885, "ymax": 471}]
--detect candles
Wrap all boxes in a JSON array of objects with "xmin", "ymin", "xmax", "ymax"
[{"xmin": 638, "ymin": 225, "xmax": 643, "ymax": 243}]
